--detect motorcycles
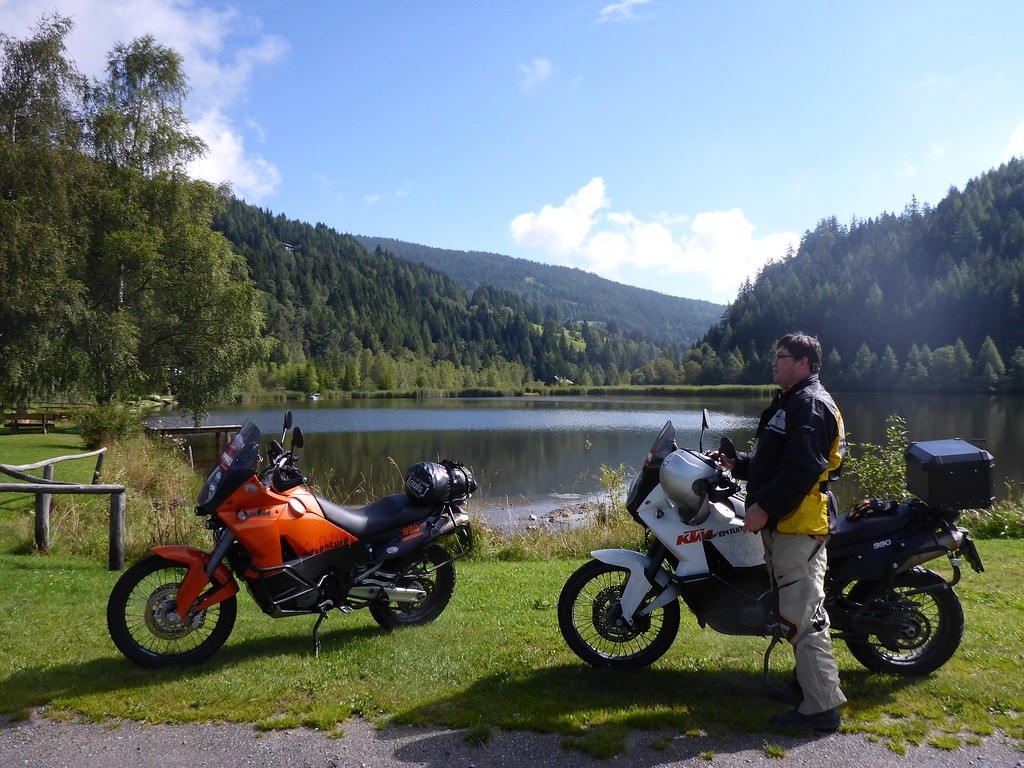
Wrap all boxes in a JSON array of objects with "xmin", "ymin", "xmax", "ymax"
[
  {"xmin": 557, "ymin": 409, "xmax": 997, "ymax": 679},
  {"xmin": 107, "ymin": 410, "xmax": 479, "ymax": 671}
]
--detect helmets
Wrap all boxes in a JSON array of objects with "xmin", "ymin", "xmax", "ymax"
[{"xmin": 659, "ymin": 448, "xmax": 718, "ymax": 510}]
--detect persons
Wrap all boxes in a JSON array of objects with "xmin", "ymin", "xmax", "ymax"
[{"xmin": 707, "ymin": 333, "xmax": 849, "ymax": 733}]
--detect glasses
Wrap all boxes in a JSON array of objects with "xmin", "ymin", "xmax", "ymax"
[{"xmin": 775, "ymin": 354, "xmax": 793, "ymax": 361}]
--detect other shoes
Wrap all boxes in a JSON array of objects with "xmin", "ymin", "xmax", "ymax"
[
  {"xmin": 771, "ymin": 708, "xmax": 840, "ymax": 733},
  {"xmin": 772, "ymin": 680, "xmax": 804, "ymax": 706}
]
